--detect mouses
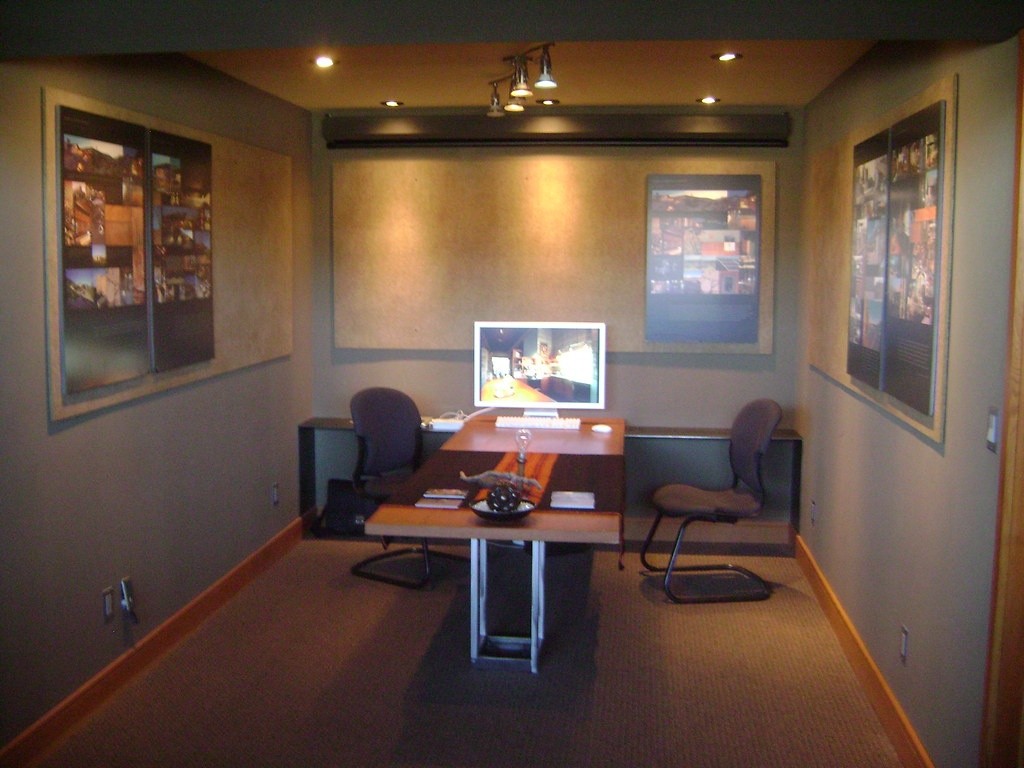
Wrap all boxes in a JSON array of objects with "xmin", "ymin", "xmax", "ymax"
[{"xmin": 591, "ymin": 424, "xmax": 612, "ymax": 433}]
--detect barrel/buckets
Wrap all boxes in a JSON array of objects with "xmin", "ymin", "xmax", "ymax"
[{"xmin": 485, "ymin": 541, "xmax": 593, "ymax": 631}]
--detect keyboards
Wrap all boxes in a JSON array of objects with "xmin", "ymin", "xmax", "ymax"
[{"xmin": 494, "ymin": 416, "xmax": 580, "ymax": 429}]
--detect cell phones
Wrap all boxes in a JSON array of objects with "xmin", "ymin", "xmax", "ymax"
[
  {"xmin": 423, "ymin": 489, "xmax": 469, "ymax": 499},
  {"xmin": 415, "ymin": 498, "xmax": 464, "ymax": 509}
]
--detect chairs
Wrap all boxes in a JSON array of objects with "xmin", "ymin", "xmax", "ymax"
[
  {"xmin": 350, "ymin": 386, "xmax": 471, "ymax": 590},
  {"xmin": 641, "ymin": 396, "xmax": 784, "ymax": 604}
]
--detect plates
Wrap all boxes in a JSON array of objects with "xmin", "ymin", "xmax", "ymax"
[{"xmin": 469, "ymin": 498, "xmax": 536, "ymax": 522}]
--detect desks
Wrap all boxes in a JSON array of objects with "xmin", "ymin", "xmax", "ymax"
[{"xmin": 364, "ymin": 418, "xmax": 627, "ymax": 674}]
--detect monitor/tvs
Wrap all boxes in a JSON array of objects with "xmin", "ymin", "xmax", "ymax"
[{"xmin": 474, "ymin": 321, "xmax": 606, "ymax": 417}]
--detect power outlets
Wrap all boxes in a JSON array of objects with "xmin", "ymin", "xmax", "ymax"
[{"xmin": 101, "ymin": 585, "xmax": 114, "ymax": 625}]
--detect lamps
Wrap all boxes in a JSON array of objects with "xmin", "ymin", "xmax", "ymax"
[{"xmin": 488, "ymin": 41, "xmax": 558, "ymax": 118}]
